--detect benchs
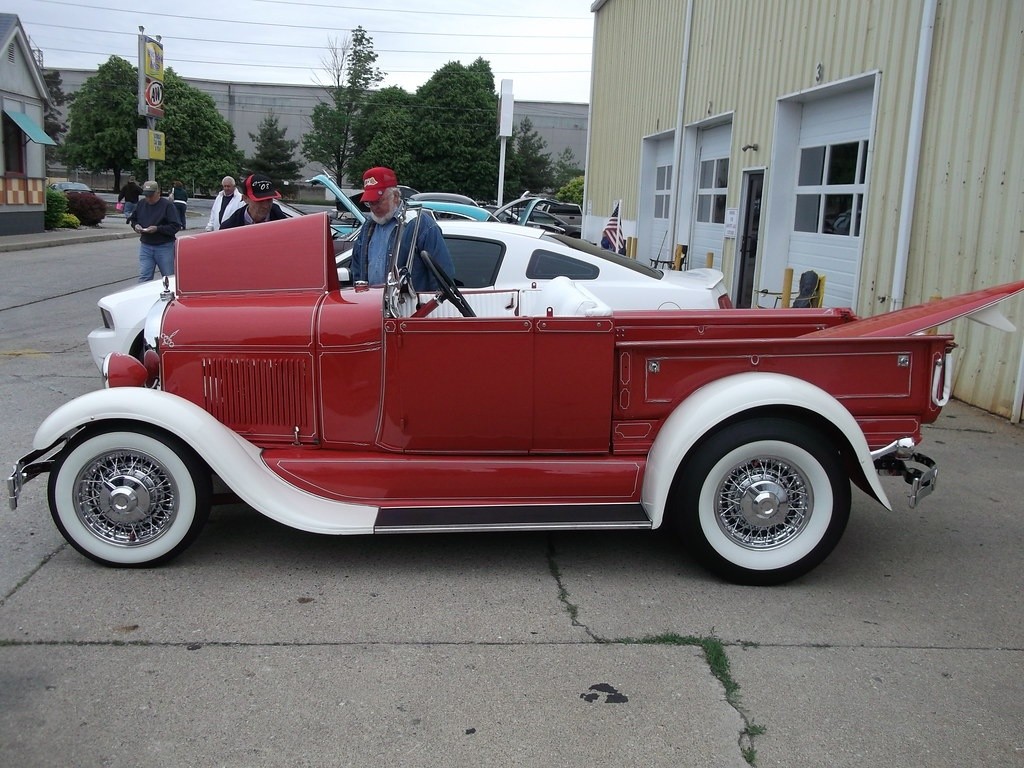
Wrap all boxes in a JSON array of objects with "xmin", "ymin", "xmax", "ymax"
[{"xmin": 530, "ymin": 275, "xmax": 611, "ymax": 316}]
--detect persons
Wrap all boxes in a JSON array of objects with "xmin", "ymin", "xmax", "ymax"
[
  {"xmin": 204, "ymin": 176, "xmax": 245, "ymax": 233},
  {"xmin": 169, "ymin": 181, "xmax": 188, "ymax": 230},
  {"xmin": 351, "ymin": 168, "xmax": 455, "ymax": 292},
  {"xmin": 130, "ymin": 181, "xmax": 181, "ymax": 284},
  {"xmin": 118, "ymin": 176, "xmax": 144, "ymax": 223},
  {"xmin": 218, "ymin": 173, "xmax": 288, "ymax": 231}
]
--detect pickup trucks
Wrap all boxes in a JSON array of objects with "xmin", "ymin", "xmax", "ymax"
[{"xmin": 7, "ymin": 211, "xmax": 1024, "ymax": 587}]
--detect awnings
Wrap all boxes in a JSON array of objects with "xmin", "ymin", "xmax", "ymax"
[{"xmin": 3, "ymin": 109, "xmax": 57, "ymax": 147}]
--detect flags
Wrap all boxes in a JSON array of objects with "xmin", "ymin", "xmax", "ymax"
[{"xmin": 600, "ymin": 204, "xmax": 626, "ymax": 255}]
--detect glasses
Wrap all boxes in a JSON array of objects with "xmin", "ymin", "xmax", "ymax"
[{"xmin": 363, "ymin": 197, "xmax": 385, "ymax": 208}]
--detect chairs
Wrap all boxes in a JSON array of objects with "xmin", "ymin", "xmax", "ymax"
[
  {"xmin": 755, "ymin": 270, "xmax": 825, "ymax": 308},
  {"xmin": 650, "ymin": 244, "xmax": 688, "ymax": 271}
]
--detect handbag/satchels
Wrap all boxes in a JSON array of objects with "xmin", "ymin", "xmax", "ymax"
[
  {"xmin": 168, "ymin": 188, "xmax": 175, "ymax": 203},
  {"xmin": 115, "ymin": 201, "xmax": 124, "ymax": 212}
]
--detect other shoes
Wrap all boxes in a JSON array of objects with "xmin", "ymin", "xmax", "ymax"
[{"xmin": 126, "ymin": 217, "xmax": 130, "ymax": 224}]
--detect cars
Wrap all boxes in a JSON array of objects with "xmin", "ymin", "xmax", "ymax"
[
  {"xmin": 49, "ymin": 182, "xmax": 94, "ymax": 194},
  {"xmin": 87, "ymin": 169, "xmax": 734, "ymax": 373}
]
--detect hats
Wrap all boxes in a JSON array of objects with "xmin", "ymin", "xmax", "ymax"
[
  {"xmin": 359, "ymin": 166, "xmax": 398, "ymax": 203},
  {"xmin": 129, "ymin": 176, "xmax": 135, "ymax": 182},
  {"xmin": 242, "ymin": 173, "xmax": 282, "ymax": 202},
  {"xmin": 141, "ymin": 180, "xmax": 159, "ymax": 196}
]
